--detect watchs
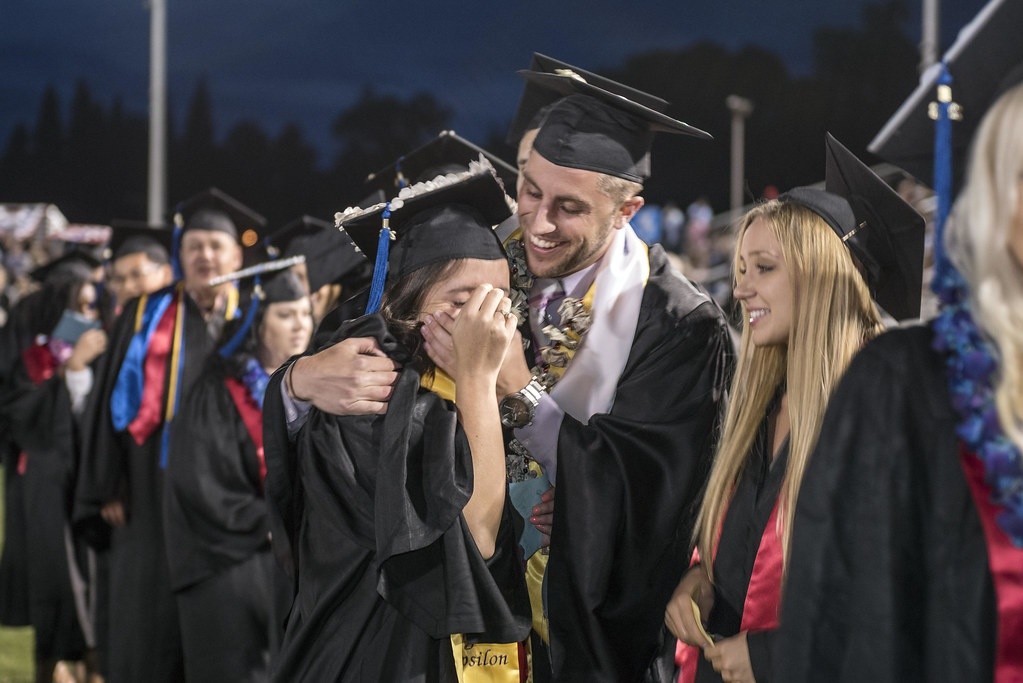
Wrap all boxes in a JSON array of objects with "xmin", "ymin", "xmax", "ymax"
[{"xmin": 499, "ymin": 375, "xmax": 546, "ymax": 429}]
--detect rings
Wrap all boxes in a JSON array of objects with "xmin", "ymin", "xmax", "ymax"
[{"xmin": 495, "ymin": 309, "xmax": 509, "ymax": 321}]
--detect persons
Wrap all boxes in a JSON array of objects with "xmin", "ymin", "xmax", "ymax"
[{"xmin": 0, "ymin": 53, "xmax": 1023, "ymax": 683}]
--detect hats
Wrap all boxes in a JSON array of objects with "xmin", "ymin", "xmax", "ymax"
[
  {"xmin": 28, "ymin": 248, "xmax": 101, "ymax": 285},
  {"xmin": 865, "ymin": 0, "xmax": 1023, "ymax": 289},
  {"xmin": 365, "ymin": 130, "xmax": 518, "ymax": 203},
  {"xmin": 507, "ymin": 51, "xmax": 670, "ymax": 149},
  {"xmin": 781, "ymin": 130, "xmax": 925, "ymax": 322},
  {"xmin": 165, "ymin": 186, "xmax": 375, "ymax": 351},
  {"xmin": 517, "ymin": 68, "xmax": 713, "ymax": 186},
  {"xmin": 333, "ymin": 152, "xmax": 519, "ymax": 316},
  {"xmin": 106, "ymin": 219, "xmax": 176, "ymax": 265}
]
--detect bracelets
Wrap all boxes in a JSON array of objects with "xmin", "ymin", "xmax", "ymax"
[{"xmin": 289, "ymin": 358, "xmax": 311, "ymax": 401}]
